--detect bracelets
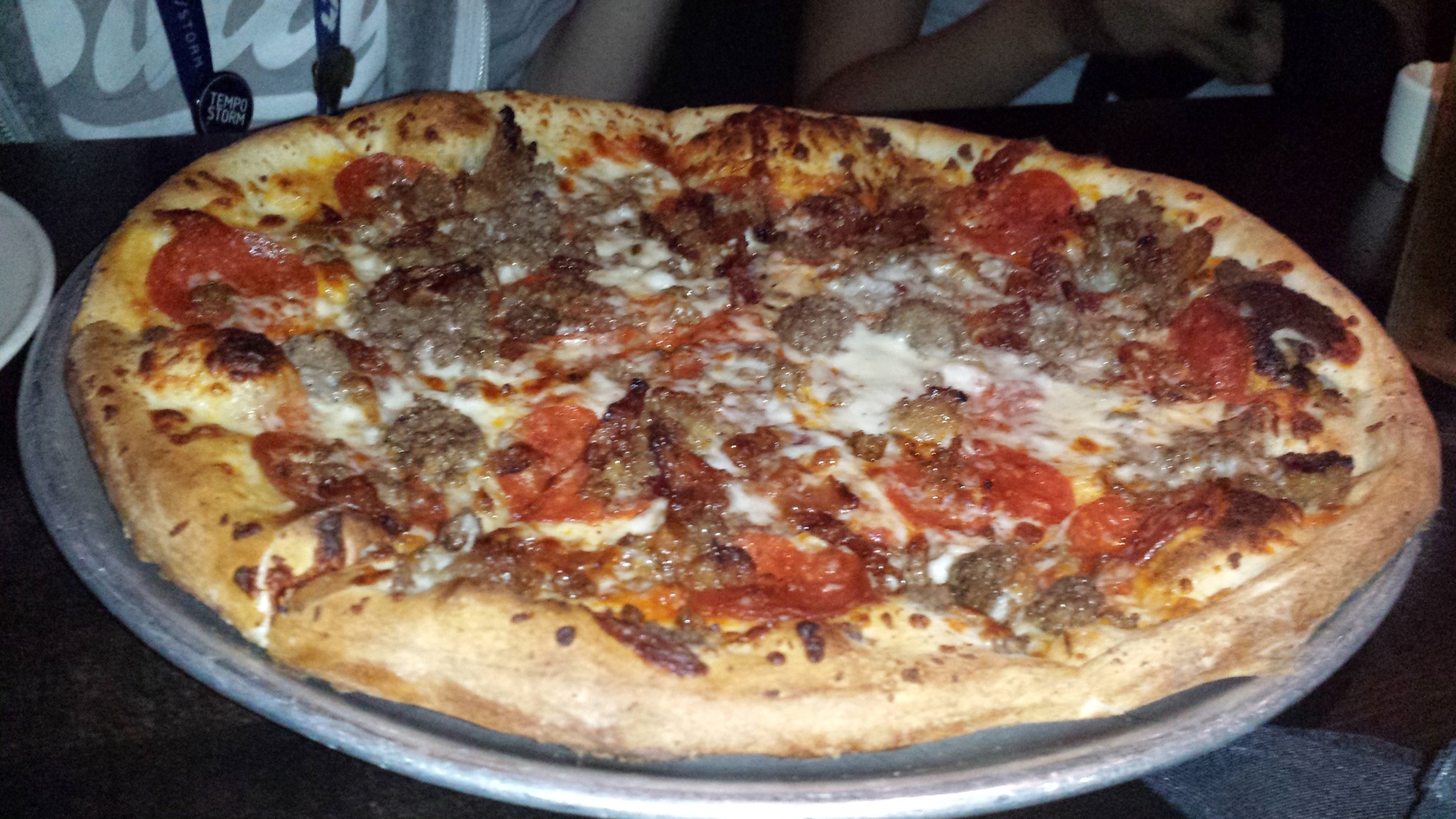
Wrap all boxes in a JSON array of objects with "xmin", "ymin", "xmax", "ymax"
[{"xmin": 1052, "ymin": 0, "xmax": 1111, "ymax": 56}]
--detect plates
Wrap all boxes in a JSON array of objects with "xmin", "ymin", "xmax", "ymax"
[
  {"xmin": 15, "ymin": 244, "xmax": 1424, "ymax": 819},
  {"xmin": 0, "ymin": 189, "xmax": 55, "ymax": 372}
]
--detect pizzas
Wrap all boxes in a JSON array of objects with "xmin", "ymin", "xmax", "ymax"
[{"xmin": 61, "ymin": 85, "xmax": 1438, "ymax": 752}]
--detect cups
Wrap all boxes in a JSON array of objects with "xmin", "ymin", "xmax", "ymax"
[{"xmin": 1380, "ymin": 62, "xmax": 1446, "ymax": 186}]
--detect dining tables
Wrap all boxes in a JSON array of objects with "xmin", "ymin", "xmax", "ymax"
[{"xmin": 0, "ymin": 93, "xmax": 1456, "ymax": 819}]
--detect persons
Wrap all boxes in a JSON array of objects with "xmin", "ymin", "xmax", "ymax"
[
  {"xmin": 801, "ymin": 0, "xmax": 1287, "ymax": 115},
  {"xmin": 0, "ymin": 0, "xmax": 667, "ymax": 149}
]
